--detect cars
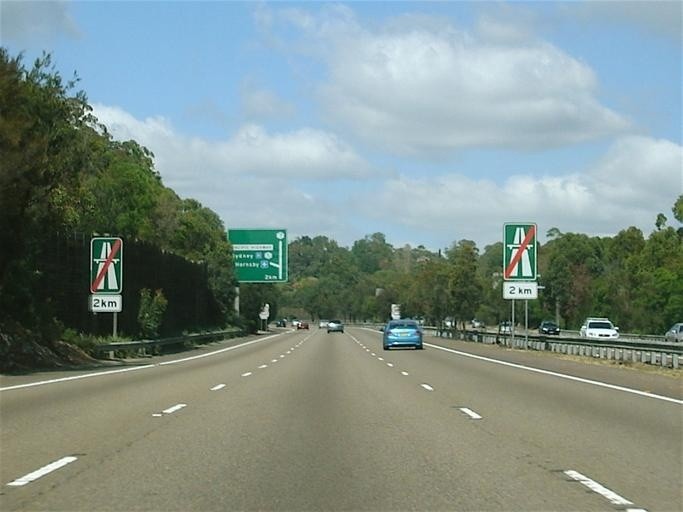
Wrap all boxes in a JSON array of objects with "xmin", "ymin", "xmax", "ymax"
[
  {"xmin": 665, "ymin": 323, "xmax": 683, "ymax": 341},
  {"xmin": 318, "ymin": 319, "xmax": 343, "ymax": 333},
  {"xmin": 472, "ymin": 319, "xmax": 486, "ymax": 328},
  {"xmin": 442, "ymin": 316, "xmax": 457, "ymax": 327},
  {"xmin": 579, "ymin": 318, "xmax": 619, "ymax": 339},
  {"xmin": 383, "ymin": 316, "xmax": 426, "ymax": 350},
  {"xmin": 276, "ymin": 319, "xmax": 308, "ymax": 329},
  {"xmin": 499, "ymin": 322, "xmax": 513, "ymax": 332},
  {"xmin": 539, "ymin": 321, "xmax": 560, "ymax": 335}
]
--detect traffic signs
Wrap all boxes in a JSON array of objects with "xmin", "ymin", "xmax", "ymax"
[{"xmin": 228, "ymin": 228, "xmax": 288, "ymax": 284}]
